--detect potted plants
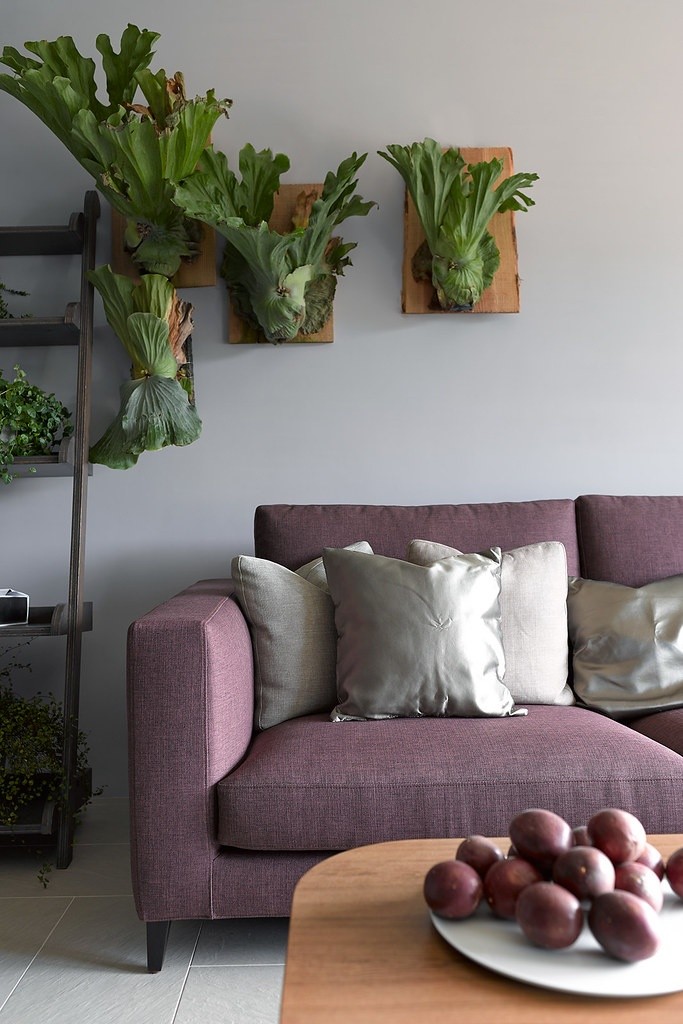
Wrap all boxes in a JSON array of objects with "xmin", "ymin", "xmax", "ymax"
[
  {"xmin": 0, "ymin": 364, "xmax": 76, "ymax": 483},
  {"xmin": 0, "ymin": 633, "xmax": 108, "ymax": 887}
]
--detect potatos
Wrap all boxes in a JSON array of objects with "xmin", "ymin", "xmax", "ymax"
[{"xmin": 422, "ymin": 808, "xmax": 683, "ymax": 965}]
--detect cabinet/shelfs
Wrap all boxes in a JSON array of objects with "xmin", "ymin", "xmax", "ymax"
[{"xmin": 0, "ymin": 188, "xmax": 103, "ymax": 871}]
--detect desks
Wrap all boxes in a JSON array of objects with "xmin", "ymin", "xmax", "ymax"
[{"xmin": 282, "ymin": 835, "xmax": 683, "ymax": 1024}]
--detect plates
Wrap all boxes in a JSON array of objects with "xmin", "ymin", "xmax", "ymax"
[{"xmin": 430, "ymin": 875, "xmax": 683, "ymax": 999}]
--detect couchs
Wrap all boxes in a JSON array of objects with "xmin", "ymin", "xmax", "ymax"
[{"xmin": 125, "ymin": 496, "xmax": 683, "ymax": 970}]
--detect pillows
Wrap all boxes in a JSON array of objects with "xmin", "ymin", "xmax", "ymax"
[
  {"xmin": 566, "ymin": 572, "xmax": 683, "ymax": 717},
  {"xmin": 232, "ymin": 541, "xmax": 377, "ymax": 731},
  {"xmin": 320, "ymin": 545, "xmax": 529, "ymax": 723},
  {"xmin": 407, "ymin": 538, "xmax": 575, "ymax": 705}
]
